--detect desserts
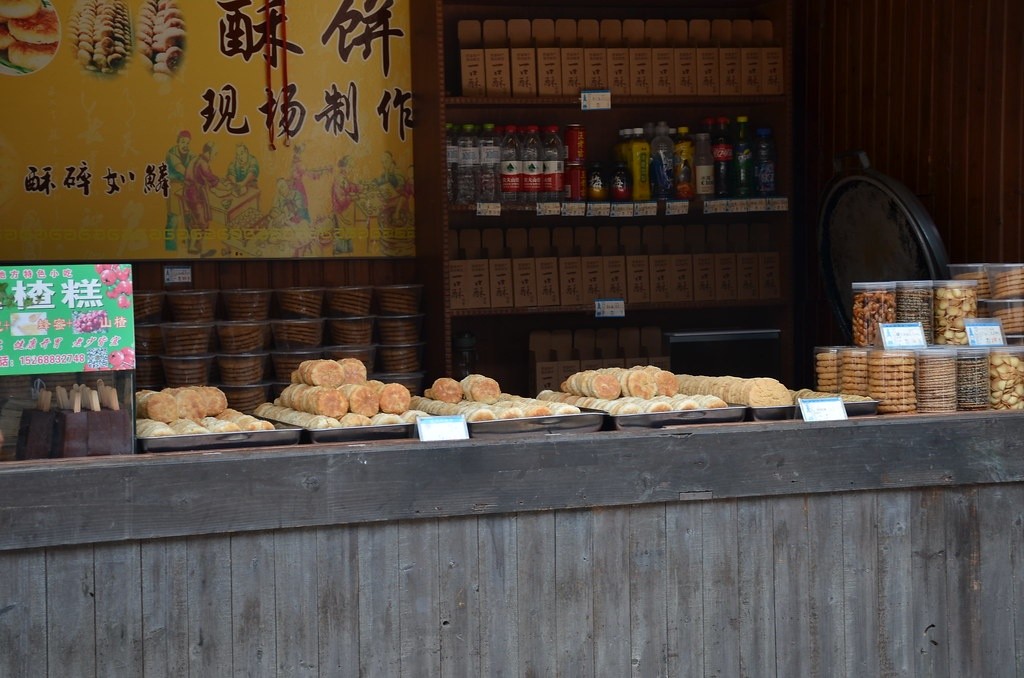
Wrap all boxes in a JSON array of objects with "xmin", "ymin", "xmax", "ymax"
[
  {"xmin": 136, "ymin": 357, "xmax": 726, "ymax": 440},
  {"xmin": 0, "ymin": 0, "xmax": 187, "ymax": 86}
]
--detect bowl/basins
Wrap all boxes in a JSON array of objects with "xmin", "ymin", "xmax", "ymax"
[
  {"xmin": 947, "ymin": 264, "xmax": 1024, "ymax": 346},
  {"xmin": 131, "ymin": 286, "xmax": 424, "ymax": 415}
]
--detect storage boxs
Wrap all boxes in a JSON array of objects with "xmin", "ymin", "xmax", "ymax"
[
  {"xmin": 457, "ymin": 20, "xmax": 784, "ymax": 98},
  {"xmin": 448, "ymin": 224, "xmax": 783, "ymax": 311},
  {"xmin": 0, "ymin": 370, "xmax": 136, "ymax": 462},
  {"xmin": 536, "ymin": 356, "xmax": 671, "ymax": 393}
]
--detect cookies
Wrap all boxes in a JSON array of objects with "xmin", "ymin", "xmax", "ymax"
[
  {"xmin": 816, "ymin": 268, "xmax": 1024, "ymax": 416},
  {"xmin": 0, "ymin": 288, "xmax": 421, "ymax": 462},
  {"xmin": 671, "ymin": 374, "xmax": 874, "ymax": 406}
]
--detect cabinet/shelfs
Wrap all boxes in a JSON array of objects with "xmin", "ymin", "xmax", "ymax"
[{"xmin": 411, "ymin": 0, "xmax": 795, "ymax": 388}]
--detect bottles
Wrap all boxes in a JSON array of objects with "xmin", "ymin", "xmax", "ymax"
[{"xmin": 447, "ymin": 115, "xmax": 780, "ymax": 200}]
